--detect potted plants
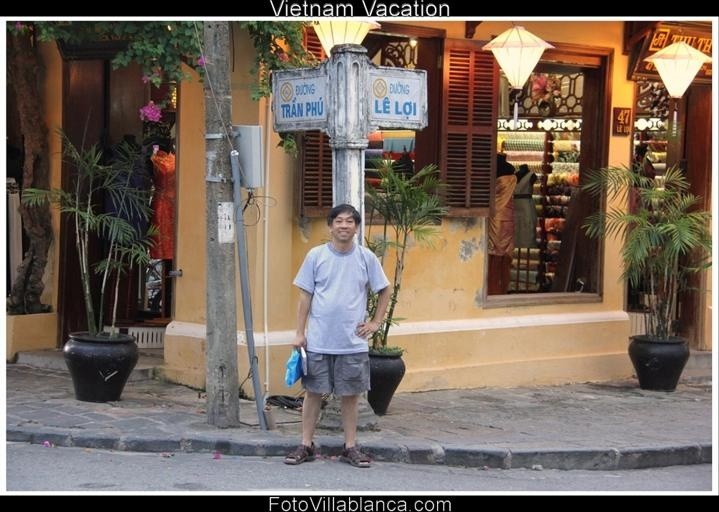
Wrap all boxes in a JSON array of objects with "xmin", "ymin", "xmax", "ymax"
[
  {"xmin": 581, "ymin": 162, "xmax": 712, "ymax": 391},
  {"xmin": 363, "ymin": 152, "xmax": 454, "ymax": 417},
  {"xmin": 25, "ymin": 109, "xmax": 140, "ymax": 403}
]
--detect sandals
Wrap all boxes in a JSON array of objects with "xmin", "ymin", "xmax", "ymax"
[
  {"xmin": 283, "ymin": 442, "xmax": 315, "ymax": 465},
  {"xmin": 340, "ymin": 442, "xmax": 371, "ymax": 467}
]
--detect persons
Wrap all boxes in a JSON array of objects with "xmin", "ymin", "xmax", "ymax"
[
  {"xmin": 489, "ymin": 153, "xmax": 514, "ymax": 293},
  {"xmin": 516, "ymin": 165, "xmax": 536, "ymax": 249},
  {"xmin": 106, "ymin": 134, "xmax": 152, "ymax": 243},
  {"xmin": 285, "ymin": 203, "xmax": 392, "ymax": 468},
  {"xmin": 630, "ymin": 145, "xmax": 657, "ymax": 237},
  {"xmin": 146, "ymin": 133, "xmax": 175, "ymax": 259}
]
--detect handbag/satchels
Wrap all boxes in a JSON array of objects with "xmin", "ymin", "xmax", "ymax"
[{"xmin": 284, "ymin": 349, "xmax": 301, "ymax": 390}]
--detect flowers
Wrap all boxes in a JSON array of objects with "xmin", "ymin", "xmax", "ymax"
[
  {"xmin": 140, "ymin": 66, "xmax": 160, "ymax": 86},
  {"xmin": 141, "ymin": 100, "xmax": 162, "ymax": 123},
  {"xmin": 198, "ymin": 56, "xmax": 205, "ymax": 66}
]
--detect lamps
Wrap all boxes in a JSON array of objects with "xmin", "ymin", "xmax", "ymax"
[
  {"xmin": 305, "ymin": 21, "xmax": 383, "ymax": 65},
  {"xmin": 477, "ymin": 23, "xmax": 555, "ymax": 90},
  {"xmin": 642, "ymin": 39, "xmax": 711, "ymax": 105}
]
